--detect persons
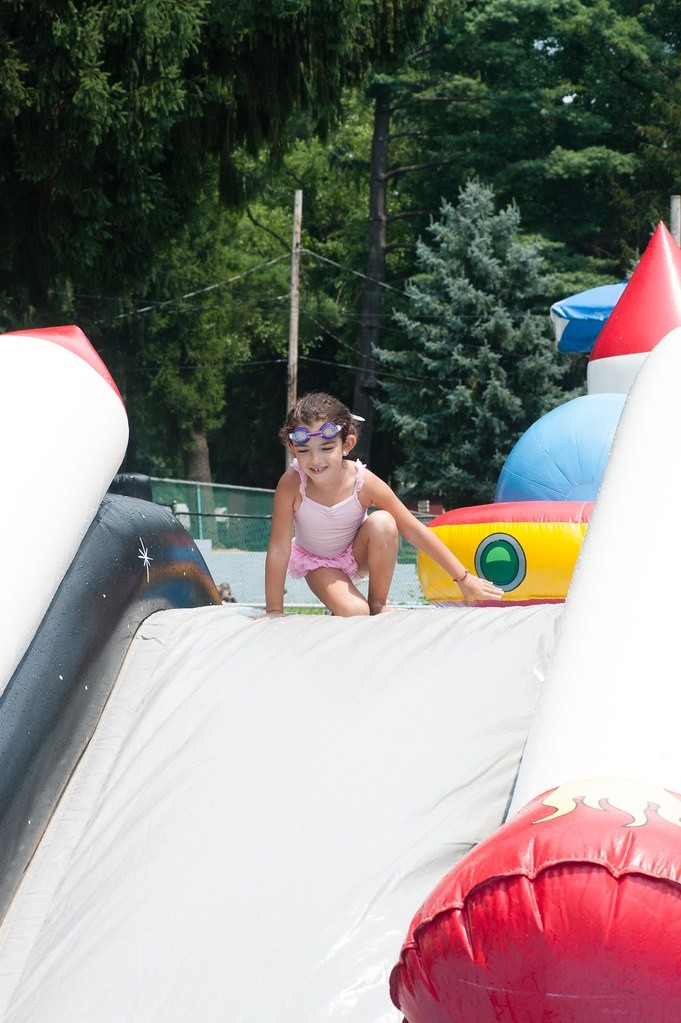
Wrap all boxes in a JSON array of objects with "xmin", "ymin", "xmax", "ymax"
[
  {"xmin": 249, "ymin": 393, "xmax": 504, "ymax": 620},
  {"xmin": 219, "ymin": 583, "xmax": 237, "ymax": 603}
]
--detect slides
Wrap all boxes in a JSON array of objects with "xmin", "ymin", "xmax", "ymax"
[{"xmin": 0, "ymin": 318, "xmax": 681, "ymax": 1023}]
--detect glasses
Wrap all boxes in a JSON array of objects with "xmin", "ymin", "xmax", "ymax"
[{"xmin": 290, "ymin": 422, "xmax": 344, "ymax": 445}]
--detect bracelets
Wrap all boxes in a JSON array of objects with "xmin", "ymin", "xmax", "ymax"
[
  {"xmin": 453, "ymin": 570, "xmax": 468, "ymax": 582},
  {"xmin": 267, "ymin": 610, "xmax": 283, "ymax": 613}
]
